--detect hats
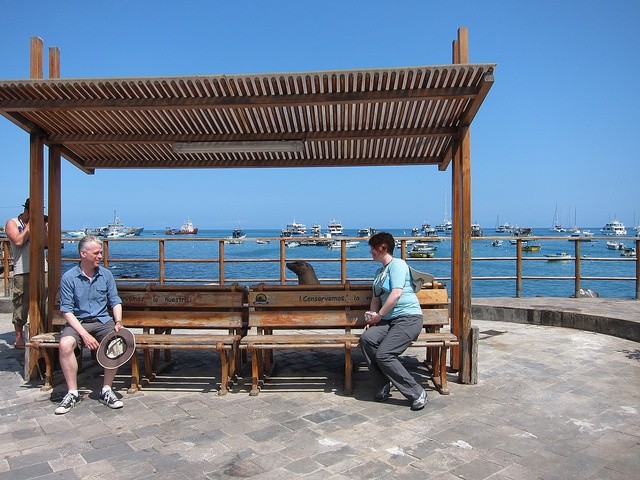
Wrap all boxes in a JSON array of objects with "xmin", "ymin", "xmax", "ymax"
[
  {"xmin": 22, "ymin": 198, "xmax": 45, "ymax": 209},
  {"xmin": 408, "ymin": 266, "xmax": 434, "ymax": 294},
  {"xmin": 96, "ymin": 327, "xmax": 135, "ymax": 370}
]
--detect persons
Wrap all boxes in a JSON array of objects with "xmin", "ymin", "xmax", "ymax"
[
  {"xmin": 359, "ymin": 232, "xmax": 429, "ymax": 411},
  {"xmin": 5, "ymin": 198, "xmax": 48, "ymax": 349},
  {"xmin": 55, "ymin": 235, "xmax": 124, "ymax": 415}
]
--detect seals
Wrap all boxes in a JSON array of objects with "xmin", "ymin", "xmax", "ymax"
[{"xmin": 286, "ymin": 261, "xmax": 320, "ymax": 285}]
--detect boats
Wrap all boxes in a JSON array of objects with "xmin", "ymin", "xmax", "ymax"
[
  {"xmin": 434, "ymin": 224, "xmax": 445, "ymax": 232},
  {"xmin": 620, "ymin": 248, "xmax": 636, "ymax": 257},
  {"xmin": 543, "ymin": 251, "xmax": 571, "ymax": 258},
  {"xmin": 67, "ymin": 232, "xmax": 86, "ymax": 237},
  {"xmin": 411, "ymin": 243, "xmax": 437, "ymax": 251},
  {"xmin": 124, "ymin": 234, "xmax": 135, "ymax": 237},
  {"xmin": 424, "ymin": 228, "xmax": 438, "ymax": 237},
  {"xmin": 583, "ymin": 230, "xmax": 594, "ymax": 236},
  {"xmin": 409, "ymin": 252, "xmax": 434, "ymax": 257},
  {"xmin": 606, "ymin": 241, "xmax": 624, "ymax": 250},
  {"xmin": 107, "ymin": 232, "xmax": 126, "ymax": 238},
  {"xmin": 224, "ymin": 228, "xmax": 246, "ymax": 244},
  {"xmin": 521, "ymin": 246, "xmax": 542, "ymax": 252},
  {"xmin": 328, "ymin": 240, "xmax": 360, "ymax": 250}
]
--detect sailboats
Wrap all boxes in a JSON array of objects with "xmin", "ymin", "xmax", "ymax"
[{"xmin": 177, "ymin": 217, "xmax": 198, "ymax": 234}]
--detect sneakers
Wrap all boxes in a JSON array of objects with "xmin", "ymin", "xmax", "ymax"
[
  {"xmin": 54, "ymin": 392, "xmax": 82, "ymax": 414},
  {"xmin": 411, "ymin": 389, "xmax": 429, "ymax": 410},
  {"xmin": 375, "ymin": 380, "xmax": 394, "ymax": 401},
  {"xmin": 97, "ymin": 387, "xmax": 124, "ymax": 409}
]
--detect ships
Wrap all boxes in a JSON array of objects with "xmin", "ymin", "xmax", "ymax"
[
  {"xmin": 280, "ymin": 220, "xmax": 351, "ymax": 246},
  {"xmin": 445, "ymin": 222, "xmax": 452, "ymax": 232},
  {"xmin": 600, "ymin": 213, "xmax": 627, "ymax": 236},
  {"xmin": 495, "ymin": 223, "xmax": 517, "ymax": 232},
  {"xmin": 421, "ymin": 224, "xmax": 431, "ymax": 233},
  {"xmin": 554, "ymin": 225, "xmax": 566, "ymax": 232},
  {"xmin": 633, "ymin": 226, "xmax": 640, "ymax": 242},
  {"xmin": 78, "ymin": 210, "xmax": 144, "ymax": 236}
]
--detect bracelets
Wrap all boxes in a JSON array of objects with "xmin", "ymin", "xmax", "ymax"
[
  {"xmin": 379, "ymin": 312, "xmax": 384, "ymax": 318},
  {"xmin": 114, "ymin": 320, "xmax": 123, "ymax": 324}
]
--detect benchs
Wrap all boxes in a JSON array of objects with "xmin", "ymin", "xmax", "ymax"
[
  {"xmin": 55, "ymin": 282, "xmax": 249, "ymax": 373},
  {"xmin": 249, "ymin": 280, "xmax": 446, "ymax": 373},
  {"xmin": 239, "ymin": 289, "xmax": 458, "ymax": 396},
  {"xmin": 25, "ymin": 291, "xmax": 242, "ymax": 396}
]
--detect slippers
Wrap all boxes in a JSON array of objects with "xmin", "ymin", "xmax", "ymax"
[{"xmin": 14, "ymin": 341, "xmax": 26, "ymax": 351}]
